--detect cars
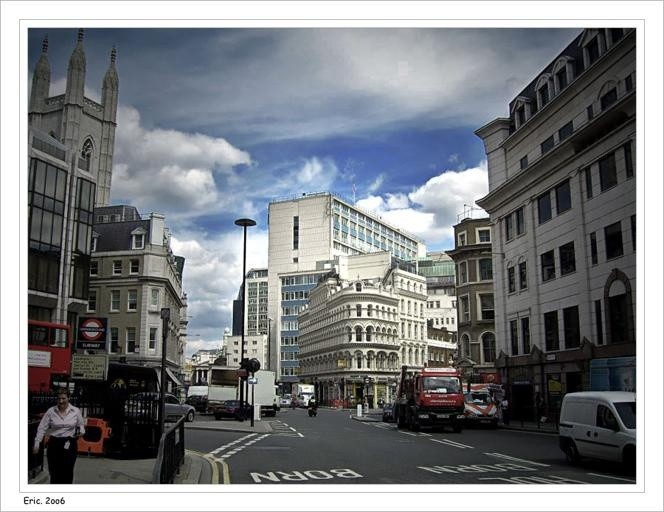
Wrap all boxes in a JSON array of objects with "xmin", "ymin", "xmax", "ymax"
[
  {"xmin": 280, "ymin": 393, "xmax": 292, "ymax": 408},
  {"xmin": 382, "ymin": 403, "xmax": 392, "ymax": 422},
  {"xmin": 120, "ymin": 392, "xmax": 195, "ymax": 424},
  {"xmin": 183, "ymin": 393, "xmax": 207, "ymax": 413},
  {"xmin": 52, "ymin": 375, "xmax": 83, "ymax": 398},
  {"xmin": 212, "ymin": 400, "xmax": 253, "ymax": 420}
]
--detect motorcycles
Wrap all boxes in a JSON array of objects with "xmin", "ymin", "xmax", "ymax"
[
  {"xmin": 291, "ymin": 400, "xmax": 297, "ymax": 410},
  {"xmin": 307, "ymin": 400, "xmax": 319, "ymax": 417}
]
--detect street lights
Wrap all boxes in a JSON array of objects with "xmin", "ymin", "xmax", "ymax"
[
  {"xmin": 448, "ymin": 353, "xmax": 454, "ymax": 368},
  {"xmin": 233, "ymin": 218, "xmax": 256, "ymax": 421}
]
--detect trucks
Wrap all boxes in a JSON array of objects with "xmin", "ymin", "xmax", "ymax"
[
  {"xmin": 71, "ymin": 363, "xmax": 157, "ymax": 408},
  {"xmin": 463, "ymin": 383, "xmax": 499, "ymax": 430},
  {"xmin": 291, "ymin": 383, "xmax": 315, "ymax": 408},
  {"xmin": 207, "ymin": 363, "xmax": 281, "ymax": 418},
  {"xmin": 390, "ymin": 362, "xmax": 470, "ymax": 433}
]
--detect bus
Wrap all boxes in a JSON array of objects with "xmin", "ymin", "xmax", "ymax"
[{"xmin": 27, "ymin": 320, "xmax": 77, "ymax": 414}]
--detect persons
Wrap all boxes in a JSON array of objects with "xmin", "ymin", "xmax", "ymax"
[
  {"xmin": 500, "ymin": 396, "xmax": 510, "ymax": 425},
  {"xmin": 307, "ymin": 395, "xmax": 318, "ymax": 409},
  {"xmin": 290, "ymin": 393, "xmax": 298, "ymax": 411},
  {"xmin": 32, "ymin": 386, "xmax": 87, "ymax": 483}
]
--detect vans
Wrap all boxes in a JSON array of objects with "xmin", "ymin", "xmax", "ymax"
[{"xmin": 558, "ymin": 391, "xmax": 636, "ymax": 475}]
